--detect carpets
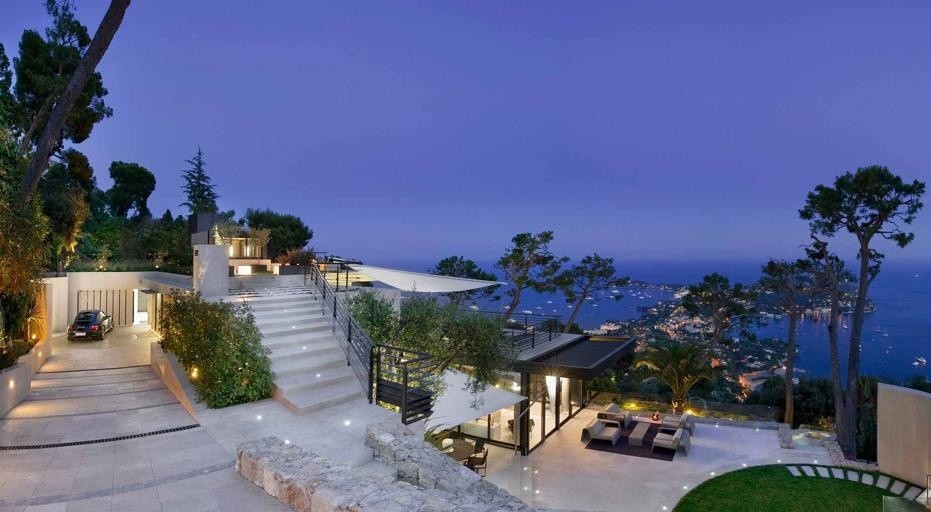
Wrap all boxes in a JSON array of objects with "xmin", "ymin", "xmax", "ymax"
[{"xmin": 583, "ymin": 420, "xmax": 676, "ymax": 462}]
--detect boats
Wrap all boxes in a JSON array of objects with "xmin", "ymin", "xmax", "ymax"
[
  {"xmin": 911, "ymin": 354, "xmax": 929, "ymax": 368},
  {"xmin": 534, "ymin": 283, "xmax": 652, "ymax": 316}
]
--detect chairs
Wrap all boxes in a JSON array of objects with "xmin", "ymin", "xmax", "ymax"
[
  {"xmin": 580, "ymin": 418, "xmax": 622, "ymax": 447},
  {"xmin": 650, "ymin": 427, "xmax": 691, "ymax": 457},
  {"xmin": 449, "ymin": 431, "xmax": 488, "ymax": 478},
  {"xmin": 596, "ymin": 403, "xmax": 632, "ymax": 429},
  {"xmin": 661, "ymin": 413, "xmax": 695, "ymax": 437}
]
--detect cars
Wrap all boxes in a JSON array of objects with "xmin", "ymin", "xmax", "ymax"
[{"xmin": 67, "ymin": 309, "xmax": 115, "ymax": 340}]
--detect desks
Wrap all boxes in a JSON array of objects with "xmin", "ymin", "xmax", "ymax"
[{"xmin": 627, "ymin": 422, "xmax": 650, "ymax": 448}]
